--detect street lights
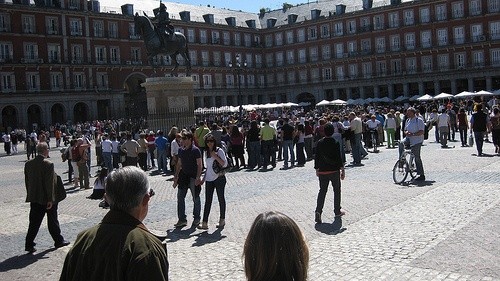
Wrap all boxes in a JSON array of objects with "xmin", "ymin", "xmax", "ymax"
[{"xmin": 228, "ymin": 53, "xmax": 248, "ymax": 119}]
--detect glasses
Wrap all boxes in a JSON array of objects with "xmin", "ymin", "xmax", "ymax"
[{"xmin": 148, "ymin": 188, "xmax": 155, "ymax": 197}]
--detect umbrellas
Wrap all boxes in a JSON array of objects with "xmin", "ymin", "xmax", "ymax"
[{"xmin": 194, "ymin": 89, "xmax": 500, "ymax": 116}]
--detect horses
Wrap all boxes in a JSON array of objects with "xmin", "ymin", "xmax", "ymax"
[{"xmin": 134, "ymin": 10, "xmax": 191, "ymax": 76}]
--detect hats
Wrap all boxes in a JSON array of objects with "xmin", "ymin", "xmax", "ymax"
[
  {"xmin": 77, "ymin": 137, "xmax": 83, "ymax": 142},
  {"xmin": 375, "ymin": 108, "xmax": 401, "ymax": 116}
]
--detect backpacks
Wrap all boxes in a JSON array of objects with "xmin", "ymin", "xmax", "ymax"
[
  {"xmin": 449, "ymin": 110, "xmax": 456, "ymax": 125},
  {"xmin": 71, "ymin": 144, "xmax": 85, "ymax": 162},
  {"xmin": 458, "ymin": 113, "xmax": 467, "ymax": 127}
]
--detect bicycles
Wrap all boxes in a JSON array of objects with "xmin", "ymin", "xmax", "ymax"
[{"xmin": 390, "ymin": 139, "xmax": 419, "ymax": 185}]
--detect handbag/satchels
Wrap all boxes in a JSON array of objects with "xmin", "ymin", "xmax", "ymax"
[
  {"xmin": 212, "ymin": 148, "xmax": 233, "ymax": 174},
  {"xmin": 469, "ymin": 135, "xmax": 473, "ymax": 147}
]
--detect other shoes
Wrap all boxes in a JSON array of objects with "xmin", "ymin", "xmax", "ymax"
[
  {"xmin": 415, "ymin": 176, "xmax": 425, "ymax": 181},
  {"xmin": 344, "ymin": 139, "xmax": 402, "ymax": 167},
  {"xmin": 335, "ymin": 211, "xmax": 345, "ymax": 217},
  {"xmin": 399, "ymin": 160, "xmax": 406, "ymax": 165},
  {"xmin": 315, "ymin": 212, "xmax": 322, "ymax": 223},
  {"xmin": 216, "ymin": 218, "xmax": 225, "ymax": 227},
  {"xmin": 173, "ymin": 219, "xmax": 187, "ymax": 226},
  {"xmin": 25, "ymin": 246, "xmax": 37, "ymax": 252},
  {"xmin": 193, "ymin": 218, "xmax": 200, "ymax": 225},
  {"xmin": 237, "ymin": 157, "xmax": 315, "ymax": 171},
  {"xmin": 436, "ymin": 134, "xmax": 500, "ymax": 157},
  {"xmin": 68, "ymin": 170, "xmax": 101, "ymax": 189},
  {"xmin": 55, "ymin": 240, "xmax": 70, "ymax": 247},
  {"xmin": 198, "ymin": 222, "xmax": 208, "ymax": 229}
]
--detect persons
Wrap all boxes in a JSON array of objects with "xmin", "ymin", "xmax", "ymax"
[
  {"xmin": 149, "ymin": 4, "xmax": 170, "ymax": 53},
  {"xmin": 314, "ymin": 123, "xmax": 345, "ymax": 223},
  {"xmin": 190, "ymin": 95, "xmax": 500, "ymax": 172},
  {"xmin": 399, "ymin": 107, "xmax": 425, "ymax": 181},
  {"xmin": 25, "ymin": 142, "xmax": 70, "ymax": 252},
  {"xmin": 242, "ymin": 212, "xmax": 309, "ymax": 281},
  {"xmin": 59, "ymin": 165, "xmax": 169, "ymax": 281},
  {"xmin": 199, "ymin": 133, "xmax": 228, "ymax": 229},
  {"xmin": 173, "ymin": 132, "xmax": 203, "ymax": 226},
  {"xmin": 2, "ymin": 116, "xmax": 189, "ymax": 189}
]
